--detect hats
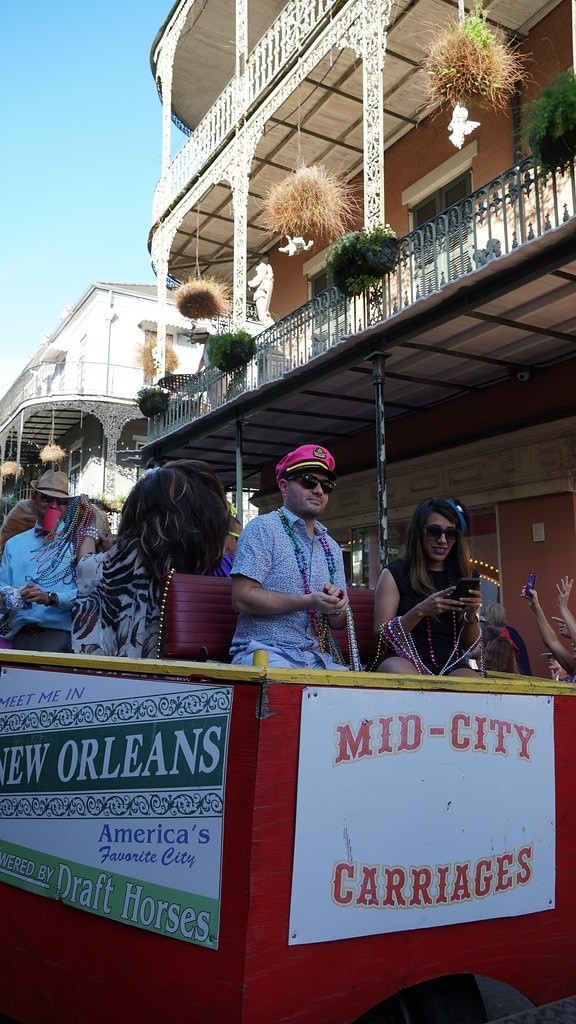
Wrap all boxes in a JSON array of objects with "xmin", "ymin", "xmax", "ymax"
[
  {"xmin": 30, "ymin": 470, "xmax": 78, "ymax": 498},
  {"xmin": 275, "ymin": 445, "xmax": 335, "ymax": 482},
  {"xmin": 540, "ymin": 653, "xmax": 552, "ymax": 661}
]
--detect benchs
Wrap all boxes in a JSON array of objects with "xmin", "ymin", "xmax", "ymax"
[{"xmin": 159, "ymin": 575, "xmax": 378, "ymax": 663}]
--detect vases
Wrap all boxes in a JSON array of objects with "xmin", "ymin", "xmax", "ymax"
[
  {"xmin": 358, "ymin": 237, "xmax": 399, "ymax": 273},
  {"xmin": 333, "ymin": 262, "xmax": 362, "ymax": 296}
]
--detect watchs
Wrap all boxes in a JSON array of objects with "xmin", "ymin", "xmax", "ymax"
[{"xmin": 45, "ymin": 592, "xmax": 57, "ymax": 607}]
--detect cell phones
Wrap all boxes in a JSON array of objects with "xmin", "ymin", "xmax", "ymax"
[
  {"xmin": 525, "ymin": 573, "xmax": 536, "ymax": 597},
  {"xmin": 449, "ymin": 578, "xmax": 480, "ymax": 608}
]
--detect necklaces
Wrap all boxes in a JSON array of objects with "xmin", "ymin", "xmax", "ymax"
[
  {"xmin": 276, "ymin": 507, "xmax": 337, "ymax": 653},
  {"xmin": 367, "ymin": 616, "xmax": 483, "ymax": 678},
  {"xmin": 24, "ymin": 492, "xmax": 93, "ymax": 588},
  {"xmin": 424, "ymin": 574, "xmax": 459, "ymax": 668},
  {"xmin": 156, "ymin": 568, "xmax": 176, "ymax": 659},
  {"xmin": 323, "ymin": 605, "xmax": 364, "ymax": 673}
]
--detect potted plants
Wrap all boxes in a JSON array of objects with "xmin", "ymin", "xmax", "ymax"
[
  {"xmin": 134, "ymin": 384, "xmax": 169, "ymax": 418},
  {"xmin": 516, "ymin": 63, "xmax": 576, "ymax": 181},
  {"xmin": 206, "ymin": 332, "xmax": 255, "ymax": 371}
]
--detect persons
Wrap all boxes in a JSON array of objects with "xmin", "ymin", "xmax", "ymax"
[
  {"xmin": 0, "ymin": 469, "xmax": 78, "ymax": 654},
  {"xmin": 195, "ymin": 341, "xmax": 228, "ymax": 409},
  {"xmin": 228, "ymin": 441, "xmax": 352, "ymax": 672},
  {"xmin": 480, "ymin": 624, "xmax": 520, "ymax": 674},
  {"xmin": 278, "ymin": 236, "xmax": 315, "ymax": 257},
  {"xmin": 207, "ymin": 516, "xmax": 243, "ymax": 577},
  {"xmin": 446, "ymin": 107, "xmax": 471, "ymax": 149},
  {"xmin": 485, "ymin": 602, "xmax": 533, "ymax": 676},
  {"xmin": 248, "ymin": 261, "xmax": 281, "ymax": 346},
  {"xmin": 67, "ymin": 460, "xmax": 228, "ymax": 660},
  {"xmin": 520, "ymin": 574, "xmax": 576, "ymax": 683},
  {"xmin": 371, "ymin": 497, "xmax": 486, "ymax": 678}
]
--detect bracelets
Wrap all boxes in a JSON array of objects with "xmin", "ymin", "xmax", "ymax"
[
  {"xmin": 85, "ymin": 526, "xmax": 99, "ymax": 543},
  {"xmin": 464, "ymin": 611, "xmax": 480, "ymax": 624}
]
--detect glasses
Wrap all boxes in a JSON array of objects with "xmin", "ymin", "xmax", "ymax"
[
  {"xmin": 285, "ymin": 475, "xmax": 336, "ymax": 493},
  {"xmin": 423, "ymin": 525, "xmax": 461, "ymax": 543},
  {"xmin": 36, "ymin": 490, "xmax": 68, "ymax": 505},
  {"xmin": 225, "ymin": 530, "xmax": 240, "ymax": 542}
]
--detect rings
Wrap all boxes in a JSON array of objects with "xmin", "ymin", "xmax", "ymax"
[{"xmin": 336, "ymin": 609, "xmax": 340, "ymax": 613}]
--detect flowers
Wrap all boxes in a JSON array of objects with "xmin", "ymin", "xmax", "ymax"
[{"xmin": 324, "ymin": 220, "xmax": 400, "ymax": 296}]
